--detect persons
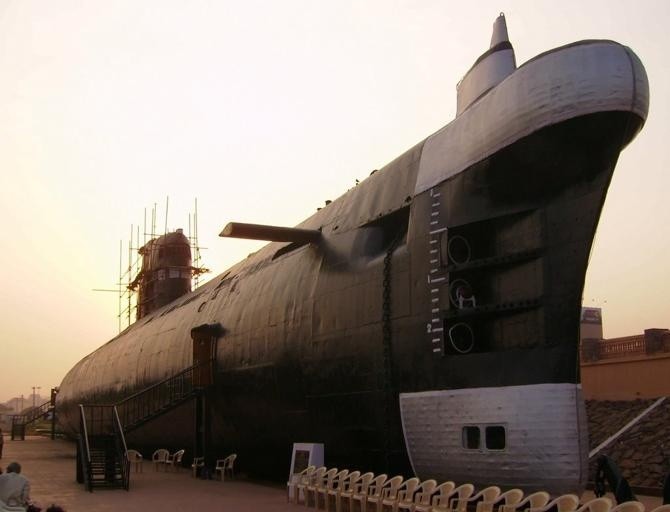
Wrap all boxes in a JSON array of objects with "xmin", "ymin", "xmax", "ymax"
[{"xmin": 0, "ymin": 461, "xmax": 31, "ymax": 511}]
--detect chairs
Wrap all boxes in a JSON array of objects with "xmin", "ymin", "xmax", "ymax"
[
  {"xmin": 152, "ymin": 449, "xmax": 169, "ymax": 472},
  {"xmin": 215, "ymin": 453, "xmax": 237, "ymax": 481},
  {"xmin": 165, "ymin": 450, "xmax": 184, "ymax": 473},
  {"xmin": 125, "ymin": 450, "xmax": 144, "ymax": 474},
  {"xmin": 286, "ymin": 465, "xmax": 670, "ymax": 512}
]
